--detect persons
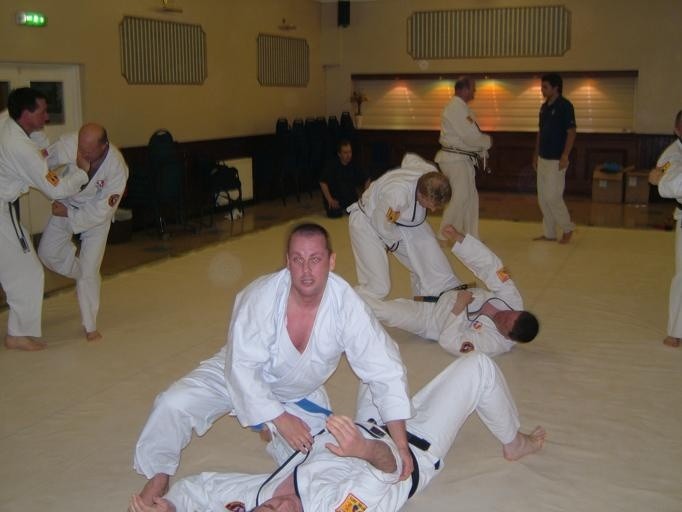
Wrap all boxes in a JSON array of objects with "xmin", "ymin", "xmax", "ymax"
[
  {"xmin": 128, "ymin": 222, "xmax": 415, "ymax": 512},
  {"xmin": 128, "ymin": 349, "xmax": 546, "ymax": 512},
  {"xmin": 36, "ymin": 123, "xmax": 130, "ymax": 342},
  {"xmin": 658, "ymin": 161, "xmax": 682, "ymax": 346},
  {"xmin": 319, "ymin": 141, "xmax": 371, "ymax": 218},
  {"xmin": 0, "ymin": 87, "xmax": 91, "ymax": 351},
  {"xmin": 354, "ymin": 225, "xmax": 539, "ymax": 357},
  {"xmin": 1, "ymin": 83, "xmax": 49, "ymax": 149},
  {"xmin": 436, "ymin": 76, "xmax": 493, "ymax": 247},
  {"xmin": 533, "ymin": 73, "xmax": 577, "ymax": 244},
  {"xmin": 655, "ymin": 109, "xmax": 682, "ymax": 169}
]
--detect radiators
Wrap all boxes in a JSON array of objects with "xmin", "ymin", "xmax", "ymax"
[{"xmin": 214, "ymin": 157, "xmax": 254, "ymax": 207}]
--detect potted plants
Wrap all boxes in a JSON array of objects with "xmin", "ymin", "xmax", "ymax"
[{"xmin": 351, "ymin": 89, "xmax": 368, "ymax": 129}]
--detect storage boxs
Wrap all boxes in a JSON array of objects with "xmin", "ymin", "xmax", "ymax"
[
  {"xmin": 592, "ymin": 164, "xmax": 634, "ymax": 204},
  {"xmin": 624, "ymin": 167, "xmax": 650, "ymax": 205}
]
